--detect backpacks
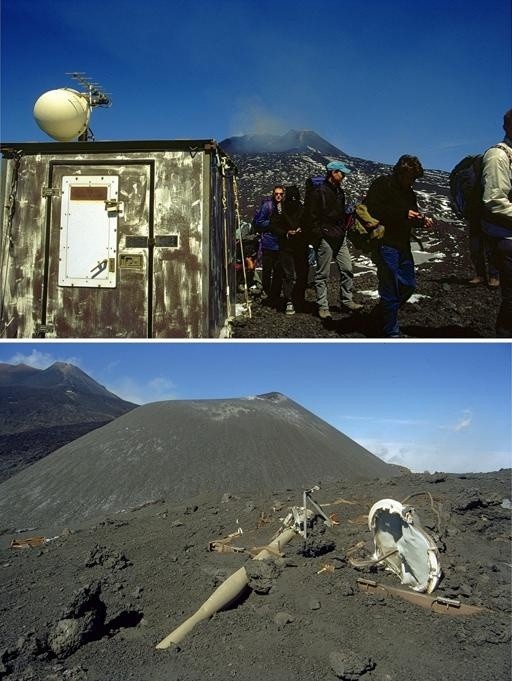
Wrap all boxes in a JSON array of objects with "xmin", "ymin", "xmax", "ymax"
[
  {"xmin": 348, "ymin": 204, "xmax": 385, "ymax": 249},
  {"xmin": 450, "ymin": 145, "xmax": 512, "ymax": 237}
]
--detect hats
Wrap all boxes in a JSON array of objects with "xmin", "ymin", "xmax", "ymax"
[
  {"xmin": 327, "ymin": 162, "xmax": 351, "ymax": 174},
  {"xmin": 393, "ymin": 155, "xmax": 423, "ymax": 177}
]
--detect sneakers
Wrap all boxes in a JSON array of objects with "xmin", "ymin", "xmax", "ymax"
[
  {"xmin": 318, "ymin": 308, "xmax": 332, "ymax": 319},
  {"xmin": 468, "ymin": 277, "xmax": 486, "ymax": 283},
  {"xmin": 342, "ymin": 302, "xmax": 363, "ymax": 312},
  {"xmin": 488, "ymin": 275, "xmax": 500, "ymax": 286},
  {"xmin": 286, "ymin": 302, "xmax": 295, "ymax": 315}
]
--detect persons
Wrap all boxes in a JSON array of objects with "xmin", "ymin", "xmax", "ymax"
[
  {"xmin": 362, "ymin": 155, "xmax": 434, "ymax": 338},
  {"xmin": 469, "ymin": 223, "xmax": 501, "ymax": 288},
  {"xmin": 252, "ymin": 185, "xmax": 310, "ymax": 316},
  {"xmin": 481, "ymin": 108, "xmax": 512, "ymax": 338},
  {"xmin": 304, "ymin": 161, "xmax": 364, "ymax": 319}
]
